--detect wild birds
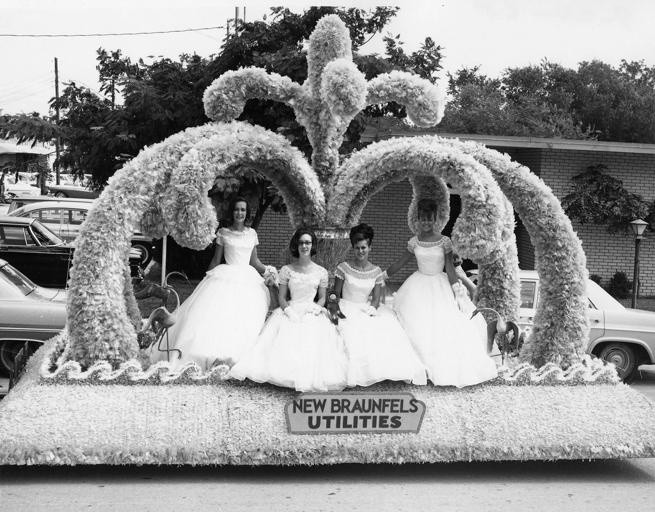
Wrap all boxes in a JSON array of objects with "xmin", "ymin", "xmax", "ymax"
[
  {"xmin": 138, "ymin": 271, "xmax": 193, "ymax": 362},
  {"xmin": 469, "ymin": 306, "xmax": 521, "ymax": 365}
]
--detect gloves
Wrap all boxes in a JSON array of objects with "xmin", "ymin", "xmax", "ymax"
[
  {"xmin": 367, "ymin": 306, "xmax": 377, "ymax": 316},
  {"xmin": 283, "ymin": 307, "xmax": 298, "ymax": 320},
  {"xmin": 313, "ymin": 304, "xmax": 321, "ymax": 315},
  {"xmin": 453, "ymin": 283, "xmax": 470, "ymax": 314}
]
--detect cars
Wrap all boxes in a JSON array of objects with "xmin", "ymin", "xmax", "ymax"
[
  {"xmin": 1, "ymin": 216, "xmax": 143, "ymax": 289},
  {"xmin": 1, "ymin": 171, "xmax": 109, "ymax": 203},
  {"xmin": 465, "ymin": 269, "xmax": 655, "ymax": 380},
  {"xmin": 7, "ymin": 196, "xmax": 155, "ymax": 268},
  {"xmin": 0, "ymin": 259, "xmax": 69, "ymax": 376}
]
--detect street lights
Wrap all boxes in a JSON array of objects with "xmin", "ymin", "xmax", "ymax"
[{"xmin": 630, "ymin": 213, "xmax": 647, "ymax": 308}]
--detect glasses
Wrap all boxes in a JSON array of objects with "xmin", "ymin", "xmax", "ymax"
[{"xmin": 295, "ymin": 240, "xmax": 312, "ymax": 245}]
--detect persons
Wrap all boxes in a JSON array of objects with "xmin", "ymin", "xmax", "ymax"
[
  {"xmin": 333, "ymin": 223, "xmax": 428, "ymax": 389},
  {"xmin": 382, "ymin": 198, "xmax": 499, "ymax": 388},
  {"xmin": 144, "ymin": 196, "xmax": 279, "ymax": 374},
  {"xmin": 227, "ymin": 227, "xmax": 349, "ymax": 394}
]
